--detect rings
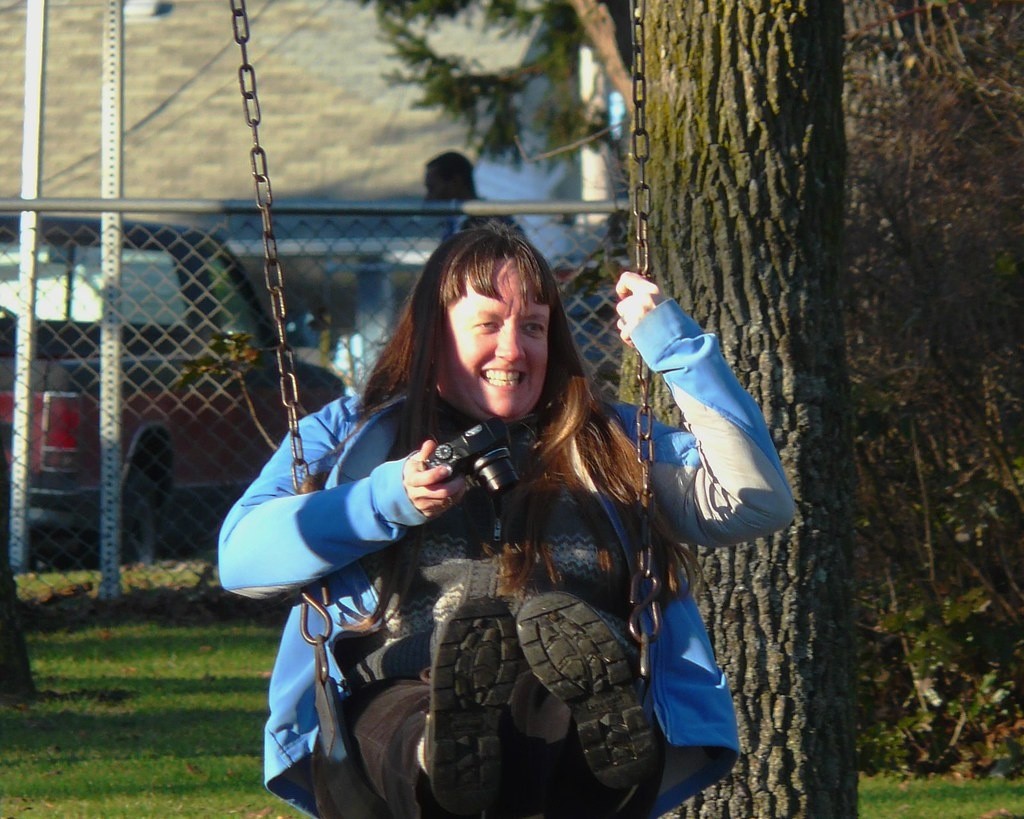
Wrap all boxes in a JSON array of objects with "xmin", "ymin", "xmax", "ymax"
[{"xmin": 443, "ymin": 496, "xmax": 455, "ymax": 509}]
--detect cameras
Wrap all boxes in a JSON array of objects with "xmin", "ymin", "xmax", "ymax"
[{"xmin": 425, "ymin": 417, "xmax": 520, "ymax": 496}]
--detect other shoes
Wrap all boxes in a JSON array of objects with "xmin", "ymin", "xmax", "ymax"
[
  {"xmin": 514, "ymin": 590, "xmax": 654, "ymax": 789},
  {"xmin": 425, "ymin": 593, "xmax": 519, "ymax": 817}
]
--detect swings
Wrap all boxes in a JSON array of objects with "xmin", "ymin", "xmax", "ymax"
[{"xmin": 227, "ymin": 0, "xmax": 670, "ymax": 819}]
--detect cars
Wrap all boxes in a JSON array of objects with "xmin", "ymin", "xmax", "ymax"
[
  {"xmin": 0, "ymin": 214, "xmax": 345, "ymax": 554},
  {"xmin": 219, "ymin": 234, "xmax": 444, "ymax": 384}
]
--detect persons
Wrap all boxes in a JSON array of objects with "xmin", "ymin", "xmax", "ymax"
[
  {"xmin": 216, "ymin": 228, "xmax": 797, "ymax": 819},
  {"xmin": 420, "ymin": 150, "xmax": 526, "ymax": 290}
]
